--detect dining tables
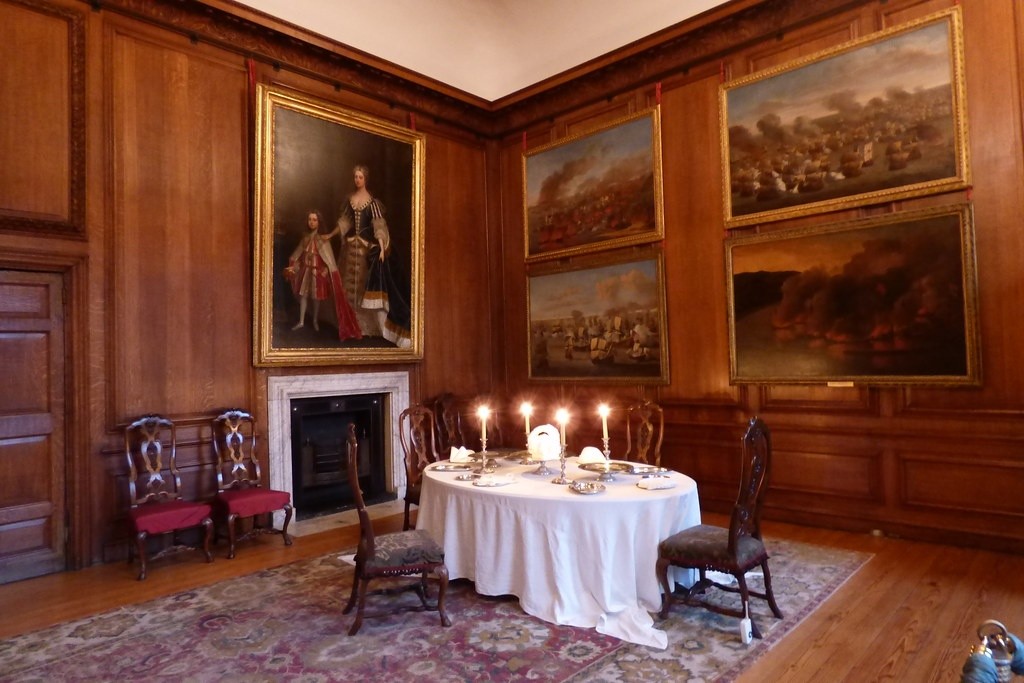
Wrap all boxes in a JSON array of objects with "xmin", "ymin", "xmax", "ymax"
[{"xmin": 415, "ymin": 457, "xmax": 701, "ymax": 649}]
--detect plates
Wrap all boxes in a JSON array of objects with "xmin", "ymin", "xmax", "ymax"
[
  {"xmin": 569, "ymin": 482, "xmax": 605, "ymax": 494},
  {"xmin": 431, "ymin": 465, "xmax": 472, "ymax": 472},
  {"xmin": 629, "ymin": 466, "xmax": 673, "ymax": 475},
  {"xmin": 456, "ymin": 474, "xmax": 481, "ymax": 480}
]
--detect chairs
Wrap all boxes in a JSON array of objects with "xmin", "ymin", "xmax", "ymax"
[
  {"xmin": 434, "ymin": 390, "xmax": 467, "ymax": 461},
  {"xmin": 622, "ymin": 398, "xmax": 664, "ymax": 467},
  {"xmin": 123, "ymin": 412, "xmax": 214, "ymax": 581},
  {"xmin": 211, "ymin": 407, "xmax": 293, "ymax": 559},
  {"xmin": 399, "ymin": 401, "xmax": 440, "ymax": 532},
  {"xmin": 655, "ymin": 416, "xmax": 784, "ymax": 640},
  {"xmin": 343, "ymin": 423, "xmax": 452, "ymax": 636}
]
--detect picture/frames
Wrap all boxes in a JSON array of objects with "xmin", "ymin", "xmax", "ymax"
[
  {"xmin": 252, "ymin": 81, "xmax": 427, "ymax": 368},
  {"xmin": 525, "ymin": 250, "xmax": 671, "ymax": 387},
  {"xmin": 723, "ymin": 202, "xmax": 983, "ymax": 387},
  {"xmin": 522, "ymin": 104, "xmax": 666, "ymax": 265},
  {"xmin": 717, "ymin": 4, "xmax": 973, "ymax": 230}
]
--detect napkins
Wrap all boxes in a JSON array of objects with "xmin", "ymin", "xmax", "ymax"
[{"xmin": 449, "ymin": 445, "xmax": 476, "ymax": 463}]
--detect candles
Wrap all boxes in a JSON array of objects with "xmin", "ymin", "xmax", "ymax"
[
  {"xmin": 602, "ymin": 407, "xmax": 608, "ymax": 438},
  {"xmin": 481, "ymin": 407, "xmax": 487, "ymax": 439},
  {"xmin": 525, "ymin": 403, "xmax": 530, "ymax": 433},
  {"xmin": 560, "ymin": 409, "xmax": 566, "ymax": 446}
]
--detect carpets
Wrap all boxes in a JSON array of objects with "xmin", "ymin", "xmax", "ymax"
[{"xmin": 0, "ymin": 536, "xmax": 876, "ymax": 683}]
